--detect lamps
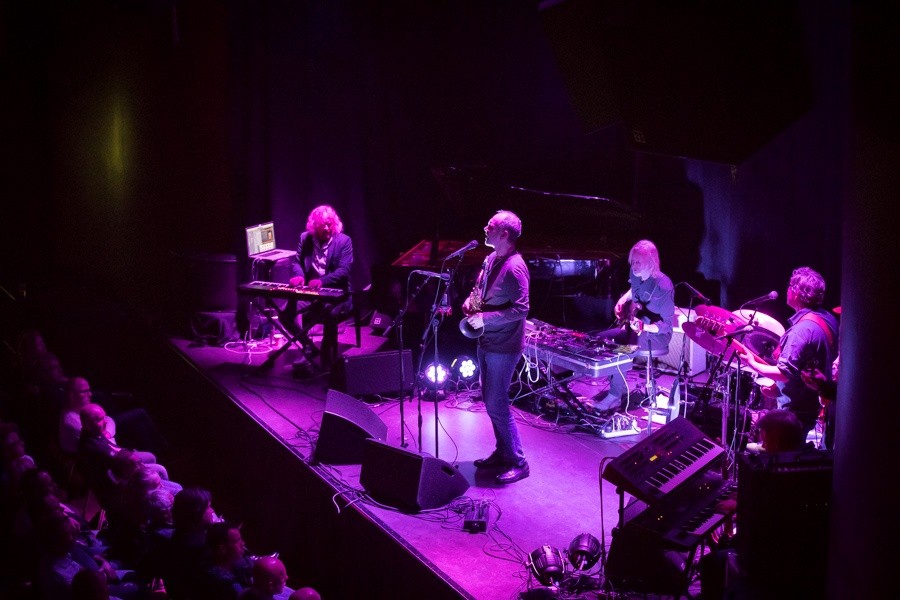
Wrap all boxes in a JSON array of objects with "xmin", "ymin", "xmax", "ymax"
[
  {"xmin": 451, "ymin": 355, "xmax": 480, "ymax": 381},
  {"xmin": 424, "ymin": 360, "xmax": 450, "ymax": 389}
]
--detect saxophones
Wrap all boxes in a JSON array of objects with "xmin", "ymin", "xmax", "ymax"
[{"xmin": 459, "ymin": 255, "xmax": 496, "ymax": 339}]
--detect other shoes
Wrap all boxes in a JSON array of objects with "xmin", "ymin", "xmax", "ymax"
[
  {"xmin": 320, "ymin": 339, "xmax": 330, "ymax": 359},
  {"xmin": 594, "ymin": 393, "xmax": 623, "ymax": 410},
  {"xmin": 302, "ymin": 345, "xmax": 319, "ymax": 358}
]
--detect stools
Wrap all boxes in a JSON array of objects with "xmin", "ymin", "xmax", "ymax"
[
  {"xmin": 302, "ymin": 301, "xmax": 361, "ymax": 358},
  {"xmin": 613, "ymin": 341, "xmax": 670, "ymax": 398}
]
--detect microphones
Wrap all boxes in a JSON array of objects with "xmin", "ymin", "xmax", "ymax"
[
  {"xmin": 444, "ymin": 240, "xmax": 478, "ymax": 261},
  {"xmin": 715, "ymin": 325, "xmax": 754, "ymax": 340},
  {"xmin": 742, "ymin": 291, "xmax": 778, "ymax": 307},
  {"xmin": 414, "ymin": 270, "xmax": 450, "ymax": 280},
  {"xmin": 684, "ymin": 281, "xmax": 712, "ymax": 306}
]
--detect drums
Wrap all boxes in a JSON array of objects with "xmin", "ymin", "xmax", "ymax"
[
  {"xmin": 706, "ymin": 358, "xmax": 762, "ymax": 387},
  {"xmin": 666, "ymin": 379, "xmax": 752, "ymax": 452}
]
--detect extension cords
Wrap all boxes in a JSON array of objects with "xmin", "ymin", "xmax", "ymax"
[{"xmin": 599, "ymin": 426, "xmax": 641, "ymax": 438}]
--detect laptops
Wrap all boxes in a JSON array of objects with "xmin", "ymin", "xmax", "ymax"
[{"xmin": 245, "ymin": 221, "xmax": 297, "ymax": 261}]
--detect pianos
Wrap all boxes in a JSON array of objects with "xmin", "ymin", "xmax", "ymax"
[
  {"xmin": 601, "ymin": 415, "xmax": 737, "ymax": 600},
  {"xmin": 236, "ymin": 278, "xmax": 345, "ymax": 381}
]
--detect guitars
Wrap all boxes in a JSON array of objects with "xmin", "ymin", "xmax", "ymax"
[
  {"xmin": 616, "ymin": 301, "xmax": 644, "ymax": 337},
  {"xmin": 694, "ymin": 315, "xmax": 782, "ymax": 398}
]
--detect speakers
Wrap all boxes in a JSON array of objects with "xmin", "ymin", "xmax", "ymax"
[
  {"xmin": 369, "ymin": 310, "xmax": 419, "ymax": 337},
  {"xmin": 632, "ymin": 328, "xmax": 707, "ymax": 377},
  {"xmin": 333, "ymin": 349, "xmax": 413, "ymax": 396},
  {"xmin": 314, "ymin": 388, "xmax": 388, "ymax": 460},
  {"xmin": 359, "ymin": 438, "xmax": 469, "ymax": 509}
]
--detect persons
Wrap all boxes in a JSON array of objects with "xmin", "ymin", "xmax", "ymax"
[
  {"xmin": 462, "ymin": 210, "xmax": 530, "ymax": 483},
  {"xmin": 0, "ymin": 331, "xmax": 321, "ymax": 600},
  {"xmin": 593, "ymin": 239, "xmax": 675, "ymax": 411},
  {"xmin": 694, "ymin": 266, "xmax": 841, "ymax": 600},
  {"xmin": 278, "ymin": 205, "xmax": 354, "ymax": 372}
]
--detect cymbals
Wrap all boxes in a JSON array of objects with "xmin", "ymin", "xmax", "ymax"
[{"xmin": 681, "ymin": 302, "xmax": 783, "ymax": 365}]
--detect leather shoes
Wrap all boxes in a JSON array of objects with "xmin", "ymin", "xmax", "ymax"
[
  {"xmin": 496, "ymin": 461, "xmax": 529, "ymax": 482},
  {"xmin": 473, "ymin": 449, "xmax": 502, "ymax": 468}
]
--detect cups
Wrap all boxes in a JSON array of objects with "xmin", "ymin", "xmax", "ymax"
[{"xmin": 274, "ymin": 333, "xmax": 284, "ymax": 345}]
